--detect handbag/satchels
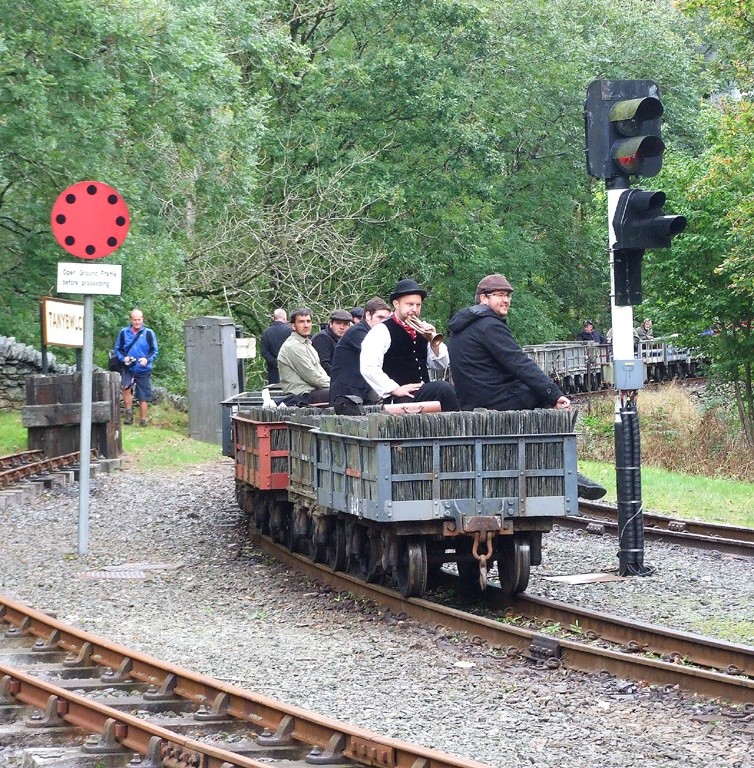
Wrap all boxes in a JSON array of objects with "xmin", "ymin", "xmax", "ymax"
[
  {"xmin": 108, "ymin": 349, "xmax": 123, "ymax": 373},
  {"xmin": 333, "ymin": 393, "xmax": 367, "ymax": 417},
  {"xmin": 383, "ymin": 400, "xmax": 442, "ymax": 414}
]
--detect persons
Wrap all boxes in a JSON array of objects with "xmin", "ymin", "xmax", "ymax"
[
  {"xmin": 277, "ymin": 310, "xmax": 332, "ymax": 404},
  {"xmin": 360, "ymin": 280, "xmax": 461, "ymax": 412},
  {"xmin": 261, "ymin": 308, "xmax": 293, "ymax": 390},
  {"xmin": 114, "ymin": 309, "xmax": 158, "ymax": 427},
  {"xmin": 448, "ymin": 274, "xmax": 607, "ymax": 500},
  {"xmin": 311, "ymin": 307, "xmax": 364, "ymax": 378},
  {"xmin": 577, "ymin": 317, "xmax": 653, "ymax": 346},
  {"xmin": 329, "ymin": 296, "xmax": 391, "ymax": 407}
]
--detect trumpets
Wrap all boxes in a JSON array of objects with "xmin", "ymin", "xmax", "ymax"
[{"xmin": 404, "ymin": 314, "xmax": 444, "ymax": 347}]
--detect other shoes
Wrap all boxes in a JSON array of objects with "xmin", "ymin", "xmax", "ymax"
[
  {"xmin": 124, "ymin": 414, "xmax": 133, "ymax": 423},
  {"xmin": 650, "ymin": 378, "xmax": 655, "ymax": 381},
  {"xmin": 140, "ymin": 419, "xmax": 148, "ymax": 426},
  {"xmin": 577, "ymin": 473, "xmax": 607, "ymax": 500}
]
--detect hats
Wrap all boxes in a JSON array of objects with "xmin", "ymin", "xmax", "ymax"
[
  {"xmin": 390, "ymin": 279, "xmax": 428, "ymax": 304},
  {"xmin": 476, "ymin": 274, "xmax": 514, "ymax": 292},
  {"xmin": 329, "ymin": 309, "xmax": 352, "ymax": 321},
  {"xmin": 350, "ymin": 307, "xmax": 364, "ymax": 317}
]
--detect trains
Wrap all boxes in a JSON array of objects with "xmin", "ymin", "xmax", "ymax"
[{"xmin": 220, "ymin": 332, "xmax": 703, "ymax": 598}]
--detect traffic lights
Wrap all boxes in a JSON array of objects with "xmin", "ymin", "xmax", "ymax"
[
  {"xmin": 612, "ymin": 188, "xmax": 688, "ymax": 250},
  {"xmin": 610, "ymin": 97, "xmax": 665, "ymax": 180}
]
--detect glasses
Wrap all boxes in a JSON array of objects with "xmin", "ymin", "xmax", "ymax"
[{"xmin": 480, "ymin": 292, "xmax": 512, "ymax": 300}]
